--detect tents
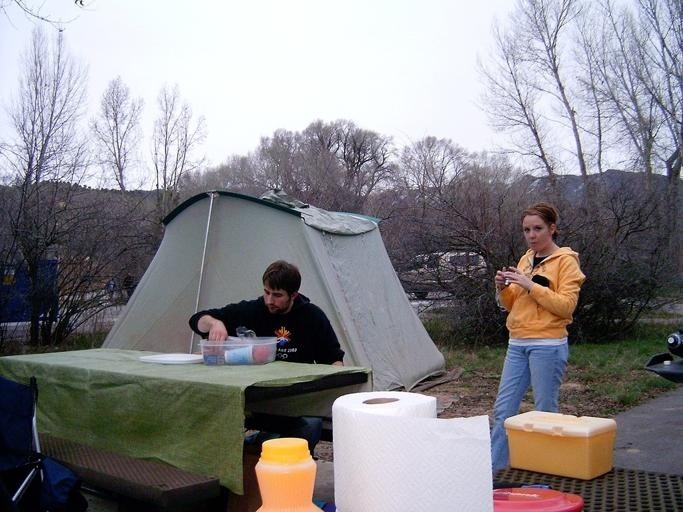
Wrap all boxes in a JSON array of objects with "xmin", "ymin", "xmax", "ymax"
[{"xmin": 100, "ymin": 188, "xmax": 446, "ymax": 391}]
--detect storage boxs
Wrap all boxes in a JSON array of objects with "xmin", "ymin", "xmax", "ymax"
[
  {"xmin": 198, "ymin": 337, "xmax": 277, "ymax": 365},
  {"xmin": 504, "ymin": 410, "xmax": 616, "ymax": 480}
]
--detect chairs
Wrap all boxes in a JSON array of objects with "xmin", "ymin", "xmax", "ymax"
[{"xmin": 0, "ymin": 376, "xmax": 88, "ymax": 511}]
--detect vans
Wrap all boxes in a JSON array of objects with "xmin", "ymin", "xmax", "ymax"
[{"xmin": 398, "ymin": 251, "xmax": 489, "ymax": 299}]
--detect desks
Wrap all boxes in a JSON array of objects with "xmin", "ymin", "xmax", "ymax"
[
  {"xmin": 0, "ymin": 348, "xmax": 368, "ymax": 512},
  {"xmin": 492, "ymin": 464, "xmax": 683, "ymax": 512}
]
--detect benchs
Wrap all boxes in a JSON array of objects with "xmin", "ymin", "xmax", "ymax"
[{"xmin": 39, "ymin": 416, "xmax": 332, "ymax": 510}]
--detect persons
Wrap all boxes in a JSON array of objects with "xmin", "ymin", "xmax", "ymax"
[
  {"xmin": 52, "ymin": 251, "xmax": 64, "ymax": 261},
  {"xmin": 491, "ymin": 202, "xmax": 586, "ymax": 473},
  {"xmin": 123, "ymin": 272, "xmax": 135, "ymax": 298},
  {"xmin": 107, "ymin": 278, "xmax": 116, "ymax": 302},
  {"xmin": 188, "ymin": 259, "xmax": 345, "ymax": 456}
]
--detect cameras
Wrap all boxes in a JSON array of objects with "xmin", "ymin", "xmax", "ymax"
[{"xmin": 503, "ymin": 272, "xmax": 514, "ymax": 286}]
